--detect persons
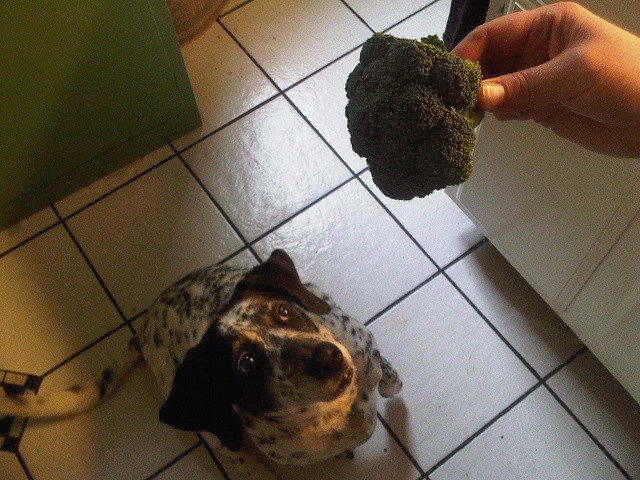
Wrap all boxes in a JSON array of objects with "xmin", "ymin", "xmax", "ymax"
[{"xmin": 448, "ymin": 0, "xmax": 640, "ymax": 158}]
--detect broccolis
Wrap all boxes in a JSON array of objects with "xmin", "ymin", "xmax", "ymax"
[{"xmin": 344, "ymin": 32, "xmax": 484, "ymax": 201}]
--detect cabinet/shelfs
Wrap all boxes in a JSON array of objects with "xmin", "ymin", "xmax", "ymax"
[
  {"xmin": 445, "ymin": 2, "xmax": 640, "ymax": 401},
  {"xmin": 1, "ymin": 0, "xmax": 203, "ymax": 231}
]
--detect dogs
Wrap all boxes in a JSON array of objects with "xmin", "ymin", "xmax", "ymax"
[{"xmin": 1, "ymin": 251, "xmax": 404, "ymax": 475}]
